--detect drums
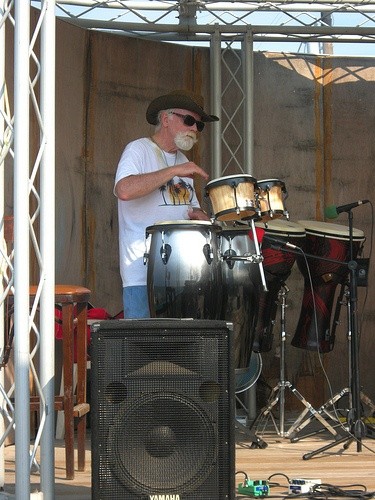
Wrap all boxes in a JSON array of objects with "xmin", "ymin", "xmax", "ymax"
[
  {"xmin": 205, "ymin": 173, "xmax": 258, "ymax": 223},
  {"xmin": 218, "ymin": 222, "xmax": 258, "ymax": 373},
  {"xmin": 251, "ymin": 176, "xmax": 286, "ymax": 223},
  {"xmin": 144, "ymin": 218, "xmax": 220, "ymax": 318},
  {"xmin": 237, "ymin": 217, "xmax": 309, "ymax": 353},
  {"xmin": 290, "ymin": 219, "xmax": 367, "ymax": 355}
]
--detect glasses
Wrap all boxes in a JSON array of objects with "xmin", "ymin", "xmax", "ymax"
[{"xmin": 167, "ymin": 112, "xmax": 205, "ymax": 131}]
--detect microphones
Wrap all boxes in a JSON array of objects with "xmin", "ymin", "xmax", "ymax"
[
  {"xmin": 325, "ymin": 200, "xmax": 369, "ymax": 219},
  {"xmin": 249, "ymin": 228, "xmax": 302, "ymax": 250}
]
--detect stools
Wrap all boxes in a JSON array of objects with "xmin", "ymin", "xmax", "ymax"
[{"xmin": 5, "ymin": 283, "xmax": 91, "ymax": 480}]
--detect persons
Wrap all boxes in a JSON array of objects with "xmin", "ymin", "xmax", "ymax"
[{"xmin": 115, "ymin": 89, "xmax": 212, "ymax": 370}]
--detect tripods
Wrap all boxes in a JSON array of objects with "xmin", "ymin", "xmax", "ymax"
[{"xmin": 249, "ymin": 210, "xmax": 375, "ymax": 460}]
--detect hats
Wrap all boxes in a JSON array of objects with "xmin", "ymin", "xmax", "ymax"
[{"xmin": 146, "ymin": 90, "xmax": 219, "ymax": 125}]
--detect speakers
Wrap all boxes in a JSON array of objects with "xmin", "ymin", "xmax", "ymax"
[{"xmin": 89, "ymin": 318, "xmax": 237, "ymax": 500}]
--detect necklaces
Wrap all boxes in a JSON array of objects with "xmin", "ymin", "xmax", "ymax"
[{"xmin": 151, "ymin": 135, "xmax": 177, "ymax": 167}]
